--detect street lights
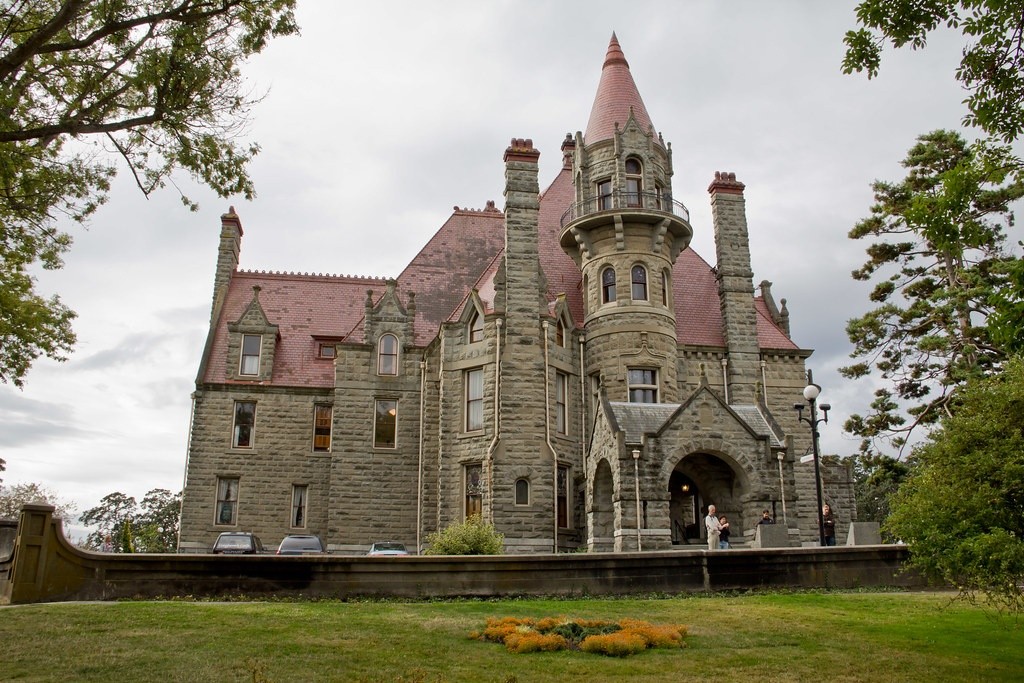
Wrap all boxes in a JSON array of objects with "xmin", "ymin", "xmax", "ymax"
[
  {"xmin": 632, "ymin": 449, "xmax": 641, "ymax": 552},
  {"xmin": 794, "ymin": 385, "xmax": 831, "ymax": 546},
  {"xmin": 777, "ymin": 451, "xmax": 788, "ymax": 524}
]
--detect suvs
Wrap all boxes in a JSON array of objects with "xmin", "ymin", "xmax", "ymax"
[
  {"xmin": 275, "ymin": 535, "xmax": 334, "ymax": 555},
  {"xmin": 213, "ymin": 531, "xmax": 268, "ymax": 554}
]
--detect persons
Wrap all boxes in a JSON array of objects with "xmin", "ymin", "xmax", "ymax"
[
  {"xmin": 716, "ymin": 515, "xmax": 730, "ymax": 550},
  {"xmin": 818, "ymin": 504, "xmax": 838, "ymax": 548},
  {"xmin": 892, "ymin": 536, "xmax": 901, "ymax": 544},
  {"xmin": 705, "ymin": 504, "xmax": 730, "ymax": 549},
  {"xmin": 754, "ymin": 508, "xmax": 773, "ymax": 528},
  {"xmin": 98, "ymin": 534, "xmax": 112, "ymax": 554}
]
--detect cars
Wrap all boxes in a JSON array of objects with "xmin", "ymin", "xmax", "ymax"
[{"xmin": 362, "ymin": 541, "xmax": 412, "ymax": 556}]
